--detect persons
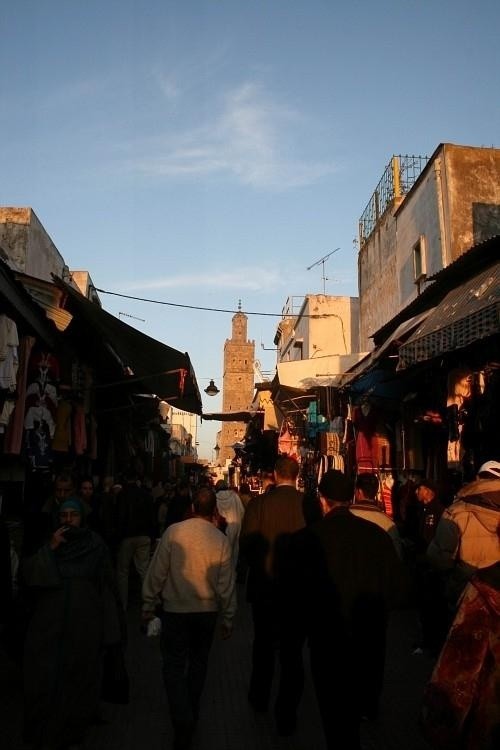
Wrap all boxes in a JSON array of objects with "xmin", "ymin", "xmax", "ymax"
[
  {"xmin": 276, "ymin": 470, "xmax": 401, "ymax": 749},
  {"xmin": 236, "ymin": 456, "xmax": 322, "ymax": 738},
  {"xmin": 118, "ymin": 469, "xmax": 156, "ymax": 633},
  {"xmin": 183, "ymin": 480, "xmax": 273, "ymax": 565},
  {"xmin": 25, "ymin": 500, "xmax": 120, "ymax": 748},
  {"xmin": 426, "ymin": 460, "xmax": 500, "ymax": 614},
  {"xmin": 142, "ymin": 488, "xmax": 238, "ymax": 747},
  {"xmin": 349, "ymin": 473, "xmax": 401, "ymax": 556},
  {"xmin": 135, "ymin": 480, "xmax": 192, "ymax": 538},
  {"xmin": 414, "ymin": 479, "xmax": 446, "ymax": 545},
  {"xmin": 78, "ymin": 475, "xmax": 122, "ymax": 559},
  {"xmin": 21, "ymin": 473, "xmax": 77, "ymax": 566}
]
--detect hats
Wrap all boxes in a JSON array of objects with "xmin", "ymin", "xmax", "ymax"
[
  {"xmin": 478, "ymin": 460, "xmax": 500, "ymax": 479},
  {"xmin": 318, "ymin": 469, "xmax": 354, "ymax": 502}
]
